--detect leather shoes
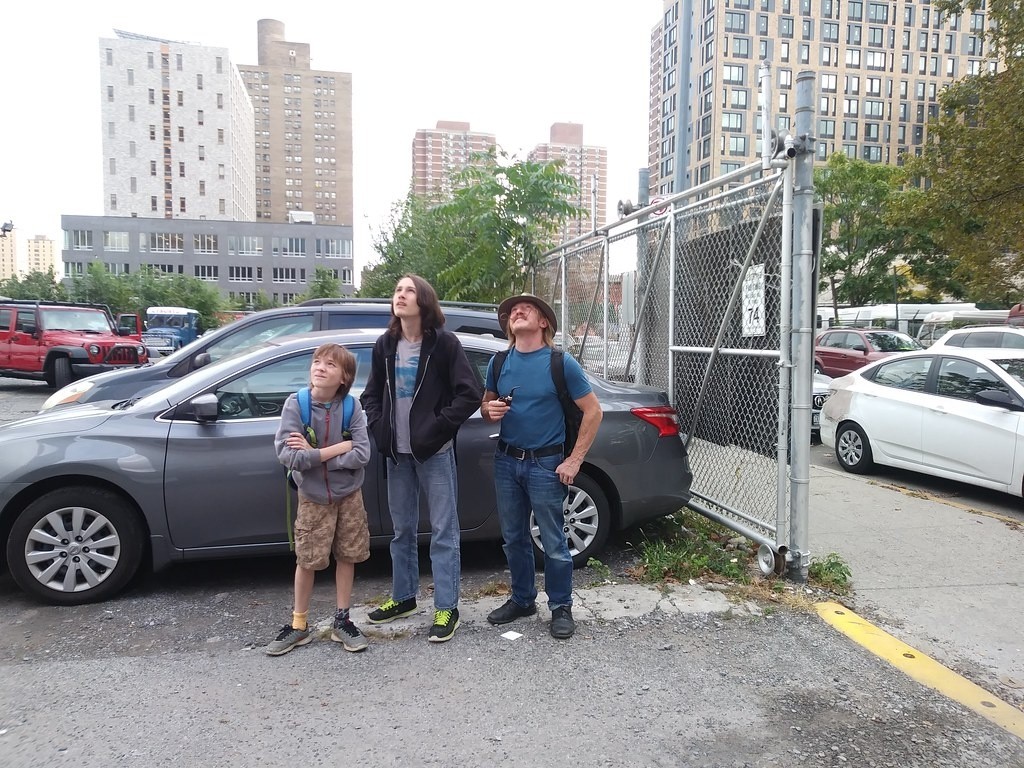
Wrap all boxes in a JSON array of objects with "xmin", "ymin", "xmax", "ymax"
[
  {"xmin": 487, "ymin": 599, "xmax": 537, "ymax": 624},
  {"xmin": 550, "ymin": 605, "xmax": 575, "ymax": 638}
]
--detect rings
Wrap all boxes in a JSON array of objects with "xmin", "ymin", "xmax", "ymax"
[{"xmin": 563, "ymin": 480, "xmax": 567, "ymax": 483}]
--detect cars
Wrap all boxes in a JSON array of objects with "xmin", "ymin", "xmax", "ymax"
[
  {"xmin": 140, "ymin": 327, "xmax": 185, "ymax": 356},
  {"xmin": 608, "ymin": 330, "xmax": 620, "ymax": 341},
  {"xmin": 916, "ymin": 310, "xmax": 1011, "ymax": 349},
  {"xmin": 0, "ymin": 326, "xmax": 694, "ymax": 607},
  {"xmin": 926, "ymin": 324, "xmax": 1024, "ymax": 350},
  {"xmin": 573, "ymin": 336, "xmax": 609, "ymax": 360},
  {"xmin": 810, "ymin": 369, "xmax": 833, "ymax": 441},
  {"xmin": 819, "ymin": 348, "xmax": 1024, "ymax": 500},
  {"xmin": 552, "ymin": 332, "xmax": 584, "ymax": 365}
]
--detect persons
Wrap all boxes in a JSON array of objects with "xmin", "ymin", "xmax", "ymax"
[
  {"xmin": 266, "ymin": 343, "xmax": 370, "ymax": 656},
  {"xmin": 360, "ymin": 274, "xmax": 482, "ymax": 643},
  {"xmin": 480, "ymin": 293, "xmax": 604, "ymax": 639}
]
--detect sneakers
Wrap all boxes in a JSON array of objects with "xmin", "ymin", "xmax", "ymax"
[
  {"xmin": 367, "ymin": 597, "xmax": 418, "ymax": 624},
  {"xmin": 266, "ymin": 623, "xmax": 312, "ymax": 655},
  {"xmin": 331, "ymin": 622, "xmax": 368, "ymax": 652},
  {"xmin": 427, "ymin": 608, "xmax": 460, "ymax": 642}
]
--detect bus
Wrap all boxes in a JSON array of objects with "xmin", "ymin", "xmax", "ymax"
[{"xmin": 143, "ymin": 304, "xmax": 202, "ymax": 348}]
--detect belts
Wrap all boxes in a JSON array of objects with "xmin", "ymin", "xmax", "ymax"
[{"xmin": 497, "ymin": 439, "xmax": 564, "ymax": 460}]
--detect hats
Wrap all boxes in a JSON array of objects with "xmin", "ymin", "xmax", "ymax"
[{"xmin": 498, "ymin": 293, "xmax": 558, "ymax": 339}]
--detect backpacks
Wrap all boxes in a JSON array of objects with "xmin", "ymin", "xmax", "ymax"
[
  {"xmin": 282, "ymin": 387, "xmax": 356, "ymax": 553},
  {"xmin": 489, "ymin": 345, "xmax": 590, "ymax": 448}
]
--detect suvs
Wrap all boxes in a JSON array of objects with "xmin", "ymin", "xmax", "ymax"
[
  {"xmin": 35, "ymin": 297, "xmax": 507, "ymax": 414},
  {"xmin": 814, "ymin": 326, "xmax": 925, "ymax": 378},
  {"xmin": 0, "ymin": 297, "xmax": 161, "ymax": 392}
]
